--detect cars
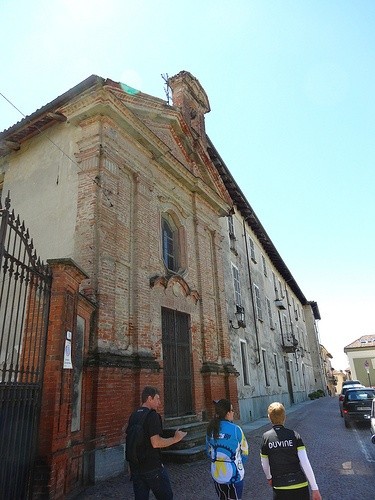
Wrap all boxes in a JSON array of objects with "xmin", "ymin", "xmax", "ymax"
[
  {"xmin": 370, "ymin": 399, "xmax": 375, "ymax": 444},
  {"xmin": 338, "ymin": 380, "xmax": 365, "ymax": 417},
  {"xmin": 339, "ymin": 388, "xmax": 375, "ymax": 429}
]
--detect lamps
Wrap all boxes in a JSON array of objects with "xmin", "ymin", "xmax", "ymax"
[{"xmin": 229, "ymin": 309, "xmax": 246, "ymax": 329}]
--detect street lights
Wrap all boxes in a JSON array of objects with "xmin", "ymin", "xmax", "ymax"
[{"xmin": 364, "ymin": 362, "xmax": 372, "ymax": 388}]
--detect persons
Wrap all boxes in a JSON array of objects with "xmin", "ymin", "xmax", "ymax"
[
  {"xmin": 205, "ymin": 399, "xmax": 249, "ymax": 500},
  {"xmin": 126, "ymin": 387, "xmax": 187, "ymax": 500},
  {"xmin": 259, "ymin": 401, "xmax": 322, "ymax": 500}
]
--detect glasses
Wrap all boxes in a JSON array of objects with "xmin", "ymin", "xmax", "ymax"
[{"xmin": 230, "ymin": 409, "xmax": 234, "ymax": 412}]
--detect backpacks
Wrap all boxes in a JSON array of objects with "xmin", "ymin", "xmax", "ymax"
[{"xmin": 125, "ymin": 409, "xmax": 156, "ymax": 466}]
